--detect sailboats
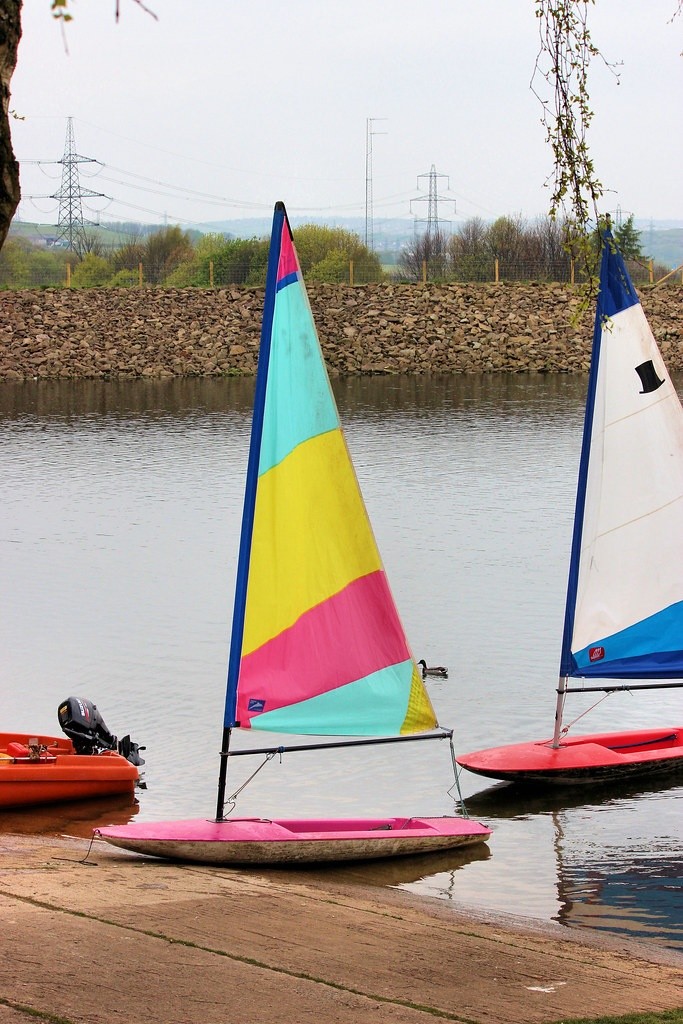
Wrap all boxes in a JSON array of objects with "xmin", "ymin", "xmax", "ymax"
[{"xmin": 82, "ymin": 197, "xmax": 494, "ymax": 867}]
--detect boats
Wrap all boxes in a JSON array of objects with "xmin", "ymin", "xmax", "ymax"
[
  {"xmin": 0, "ymin": 694, "xmax": 147, "ymax": 810},
  {"xmin": 450, "ymin": 211, "xmax": 683, "ymax": 788}
]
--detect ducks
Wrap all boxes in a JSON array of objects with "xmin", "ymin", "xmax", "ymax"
[{"xmin": 416, "ymin": 658, "xmax": 449, "ymax": 676}]
